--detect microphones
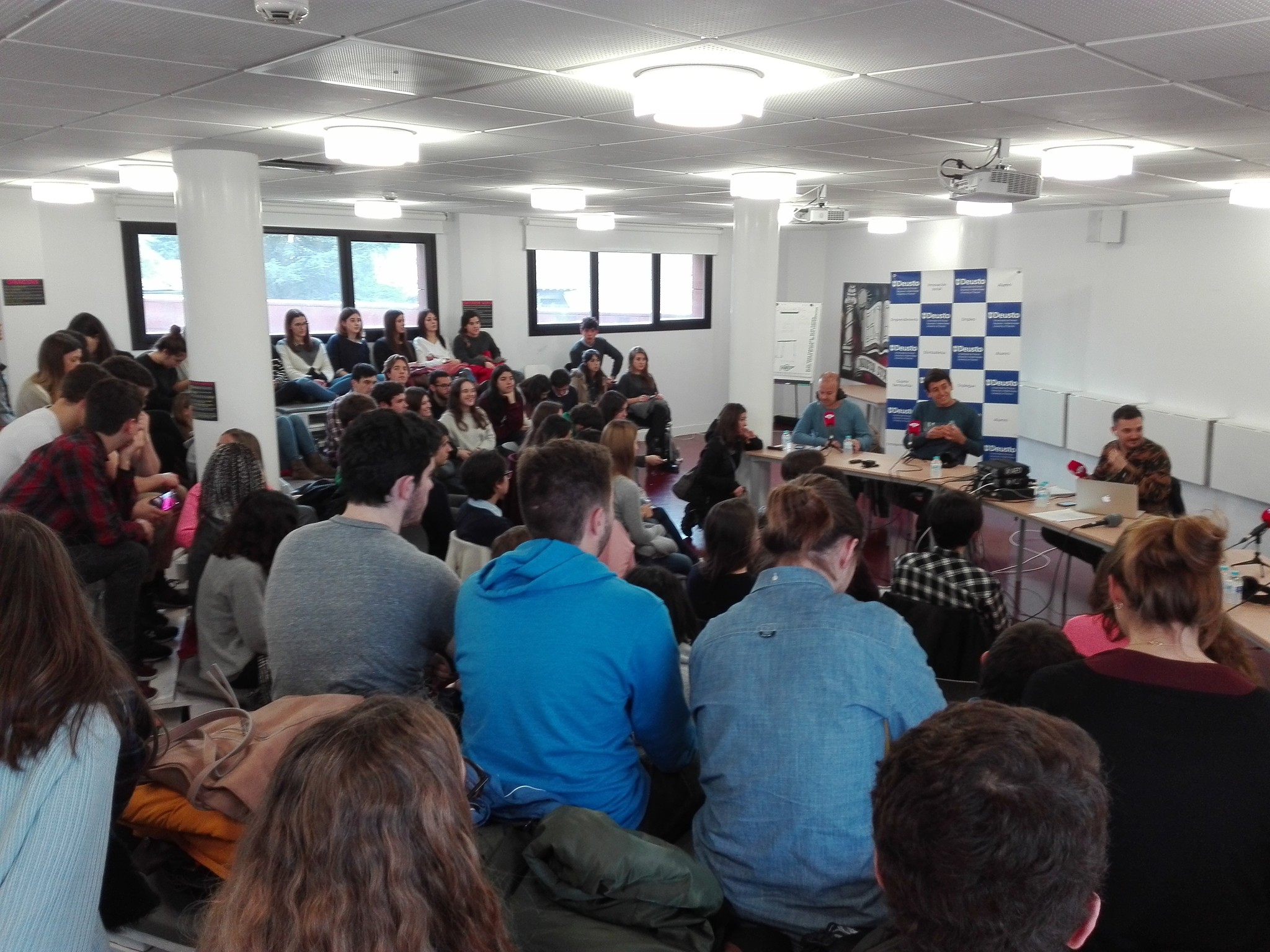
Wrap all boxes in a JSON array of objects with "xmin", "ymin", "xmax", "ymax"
[
  {"xmin": 824, "ymin": 410, "xmax": 835, "ymax": 436},
  {"xmin": 909, "ymin": 420, "xmax": 922, "ymax": 446},
  {"xmin": 1080, "ymin": 512, "xmax": 1123, "ymax": 528},
  {"xmin": 1068, "ymin": 461, "xmax": 1089, "ymax": 478},
  {"xmin": 1242, "ymin": 508, "xmax": 1270, "ymax": 542}
]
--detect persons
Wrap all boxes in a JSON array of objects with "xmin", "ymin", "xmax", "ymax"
[
  {"xmin": 903, "ymin": 368, "xmax": 983, "ymax": 467},
  {"xmin": 0, "ymin": 307, "xmax": 1270, "ymax": 952},
  {"xmin": 681, "ymin": 404, "xmax": 763, "ymax": 537},
  {"xmin": 1077, "ymin": 402, "xmax": 1174, "ymax": 518},
  {"xmin": 791, "ymin": 372, "xmax": 875, "ymax": 451},
  {"xmin": 685, "ymin": 474, "xmax": 952, "ymax": 952},
  {"xmin": 263, "ymin": 409, "xmax": 466, "ymax": 702}
]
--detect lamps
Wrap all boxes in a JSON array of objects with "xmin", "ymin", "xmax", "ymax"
[
  {"xmin": 531, "ymin": 188, "xmax": 585, "ymax": 210},
  {"xmin": 632, "ymin": 63, "xmax": 766, "ymax": 127},
  {"xmin": 119, "ymin": 163, "xmax": 177, "ymax": 192},
  {"xmin": 354, "ymin": 201, "xmax": 401, "ymax": 218},
  {"xmin": 324, "ymin": 125, "xmax": 419, "ymax": 164},
  {"xmin": 577, "ymin": 212, "xmax": 615, "ymax": 231},
  {"xmin": 1043, "ymin": 144, "xmax": 1134, "ymax": 181},
  {"xmin": 867, "ymin": 218, "xmax": 908, "ymax": 234},
  {"xmin": 957, "ymin": 200, "xmax": 1013, "ymax": 217},
  {"xmin": 730, "ymin": 172, "xmax": 797, "ymax": 198},
  {"xmin": 1230, "ymin": 183, "xmax": 1270, "ymax": 208},
  {"xmin": 31, "ymin": 181, "xmax": 95, "ymax": 204}
]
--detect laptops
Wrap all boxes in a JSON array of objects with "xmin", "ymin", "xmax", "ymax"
[{"xmin": 1076, "ymin": 479, "xmax": 1147, "ymax": 518}]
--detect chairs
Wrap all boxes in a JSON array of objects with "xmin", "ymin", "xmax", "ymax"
[{"xmin": 884, "ymin": 591, "xmax": 997, "ymax": 682}]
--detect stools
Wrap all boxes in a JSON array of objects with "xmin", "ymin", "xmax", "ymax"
[{"xmin": 636, "ymin": 422, "xmax": 674, "ymax": 464}]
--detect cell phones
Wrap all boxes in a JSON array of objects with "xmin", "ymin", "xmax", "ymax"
[
  {"xmin": 149, "ymin": 489, "xmax": 181, "ymax": 512},
  {"xmin": 1056, "ymin": 500, "xmax": 1077, "ymax": 507}
]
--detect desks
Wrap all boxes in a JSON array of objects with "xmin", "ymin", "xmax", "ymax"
[{"xmin": 747, "ymin": 384, "xmax": 1270, "ymax": 652}]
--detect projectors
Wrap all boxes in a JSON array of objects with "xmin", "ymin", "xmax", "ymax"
[
  {"xmin": 789, "ymin": 206, "xmax": 849, "ymax": 223},
  {"xmin": 949, "ymin": 168, "xmax": 1043, "ymax": 203}
]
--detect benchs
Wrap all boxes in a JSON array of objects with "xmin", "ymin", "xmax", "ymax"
[{"xmin": 271, "ymin": 342, "xmax": 376, "ymax": 431}]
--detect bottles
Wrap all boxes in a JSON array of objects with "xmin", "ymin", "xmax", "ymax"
[
  {"xmin": 781, "ymin": 430, "xmax": 793, "ymax": 454},
  {"xmin": 930, "ymin": 456, "xmax": 943, "ymax": 478},
  {"xmin": 843, "ymin": 436, "xmax": 853, "ymax": 455},
  {"xmin": 1036, "ymin": 481, "xmax": 1050, "ymax": 508},
  {"xmin": 1220, "ymin": 565, "xmax": 1243, "ymax": 604}
]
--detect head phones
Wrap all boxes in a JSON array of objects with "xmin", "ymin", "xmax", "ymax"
[
  {"xmin": 815, "ymin": 376, "xmax": 845, "ymax": 400},
  {"xmin": 1240, "ymin": 575, "xmax": 1270, "ymax": 605}
]
